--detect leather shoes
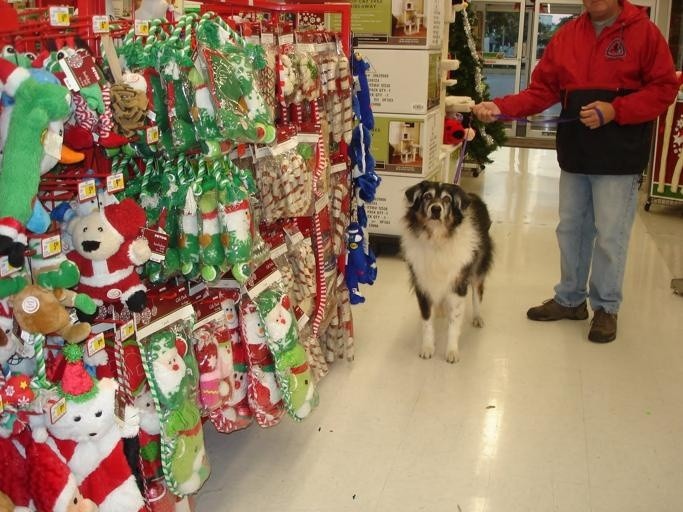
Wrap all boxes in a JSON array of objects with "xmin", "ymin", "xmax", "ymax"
[
  {"xmin": 527, "ymin": 298, "xmax": 589, "ymax": 322},
  {"xmin": 588, "ymin": 308, "xmax": 618, "ymax": 342}
]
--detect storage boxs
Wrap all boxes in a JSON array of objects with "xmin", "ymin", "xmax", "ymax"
[{"xmin": 322, "ymin": 0, "xmax": 463, "ymax": 236}]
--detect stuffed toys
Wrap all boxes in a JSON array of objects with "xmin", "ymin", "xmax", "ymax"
[
  {"xmin": 0, "ymin": 46, "xmax": 382, "ymax": 512},
  {"xmin": 444, "ymin": 112, "xmax": 476, "ymax": 143}
]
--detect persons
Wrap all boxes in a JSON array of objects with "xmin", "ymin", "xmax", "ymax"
[{"xmin": 471, "ymin": 0, "xmax": 679, "ymax": 343}]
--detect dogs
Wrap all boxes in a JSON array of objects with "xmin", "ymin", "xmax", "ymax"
[{"xmin": 400, "ymin": 180, "xmax": 496, "ymax": 365}]
